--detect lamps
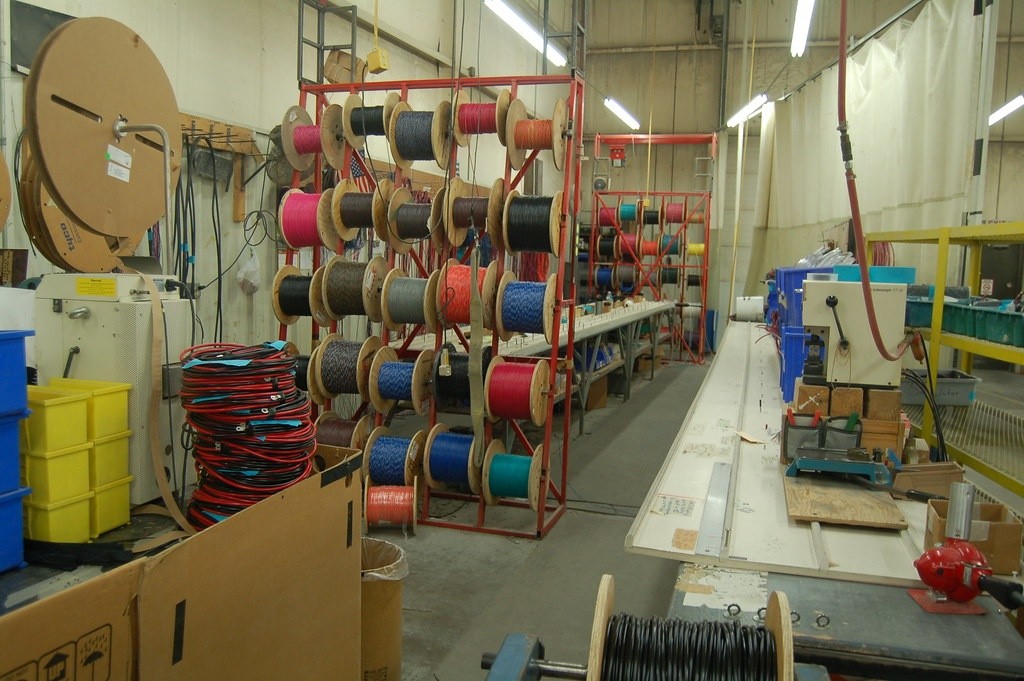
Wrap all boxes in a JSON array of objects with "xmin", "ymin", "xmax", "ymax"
[
  {"xmin": 726, "ymin": 94, "xmax": 768, "ymax": 128},
  {"xmin": 484, "ymin": 0, "xmax": 641, "ymax": 130}
]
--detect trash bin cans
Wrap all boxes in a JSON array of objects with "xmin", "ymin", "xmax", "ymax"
[{"xmin": 360, "ymin": 536, "xmax": 409, "ymax": 681}]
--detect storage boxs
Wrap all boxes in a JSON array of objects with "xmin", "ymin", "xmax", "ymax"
[
  {"xmin": 767, "ymin": 259, "xmax": 834, "ymax": 404},
  {"xmin": 0, "ymin": 330, "xmax": 135, "ymax": 574},
  {"xmin": 900, "ymin": 367, "xmax": 983, "ymax": 407},
  {"xmin": 905, "ymin": 295, "xmax": 1024, "ymax": 347}
]
